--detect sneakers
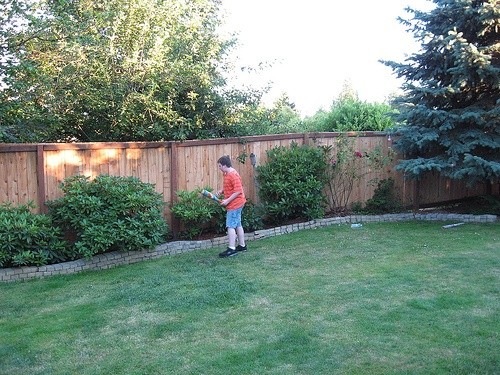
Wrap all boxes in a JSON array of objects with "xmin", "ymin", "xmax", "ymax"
[
  {"xmin": 218, "ymin": 247, "xmax": 238, "ymax": 258},
  {"xmin": 236, "ymin": 245, "xmax": 247, "ymax": 252}
]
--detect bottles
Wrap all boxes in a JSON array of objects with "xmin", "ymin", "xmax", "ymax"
[{"xmin": 351, "ymin": 223, "xmax": 362, "ymax": 228}]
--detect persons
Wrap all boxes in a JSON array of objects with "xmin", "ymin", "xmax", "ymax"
[{"xmin": 215, "ymin": 156, "xmax": 248, "ymax": 258}]
